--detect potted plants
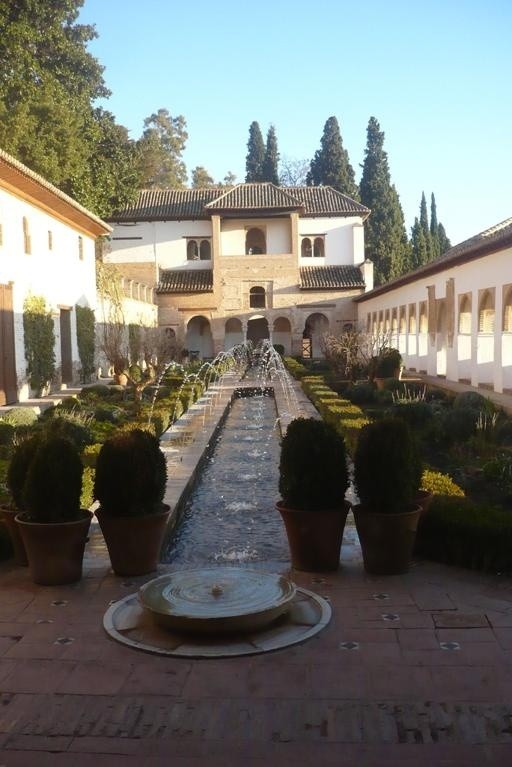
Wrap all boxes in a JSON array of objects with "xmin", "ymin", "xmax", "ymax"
[
  {"xmin": 0, "ymin": 429, "xmax": 173, "ymax": 586},
  {"xmin": 113, "ymin": 357, "xmax": 128, "ymax": 386},
  {"xmin": 373, "ymin": 347, "xmax": 403, "ymax": 392},
  {"xmin": 274, "ymin": 417, "xmax": 426, "ymax": 577}
]
